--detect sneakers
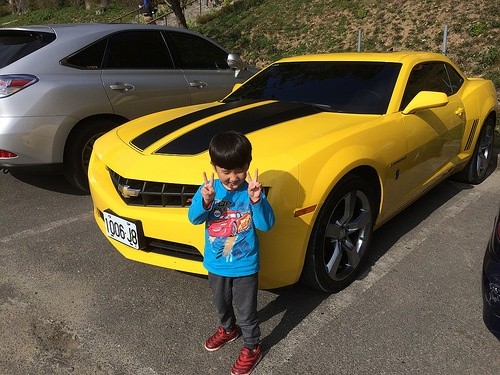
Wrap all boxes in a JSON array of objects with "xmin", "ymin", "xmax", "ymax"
[
  {"xmin": 204, "ymin": 325, "xmax": 240, "ymax": 352},
  {"xmin": 230, "ymin": 343, "xmax": 262, "ymax": 375}
]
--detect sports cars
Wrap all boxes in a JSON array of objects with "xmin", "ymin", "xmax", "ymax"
[{"xmin": 86, "ymin": 50, "xmax": 499, "ymax": 295}]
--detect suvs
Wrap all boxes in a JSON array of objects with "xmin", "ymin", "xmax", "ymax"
[{"xmin": 0, "ymin": 22, "xmax": 264, "ymax": 195}]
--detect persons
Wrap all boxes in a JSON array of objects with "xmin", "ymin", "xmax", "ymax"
[
  {"xmin": 188, "ymin": 131, "xmax": 275, "ymax": 375},
  {"xmin": 138, "ymin": 0, "xmax": 152, "ymax": 24}
]
